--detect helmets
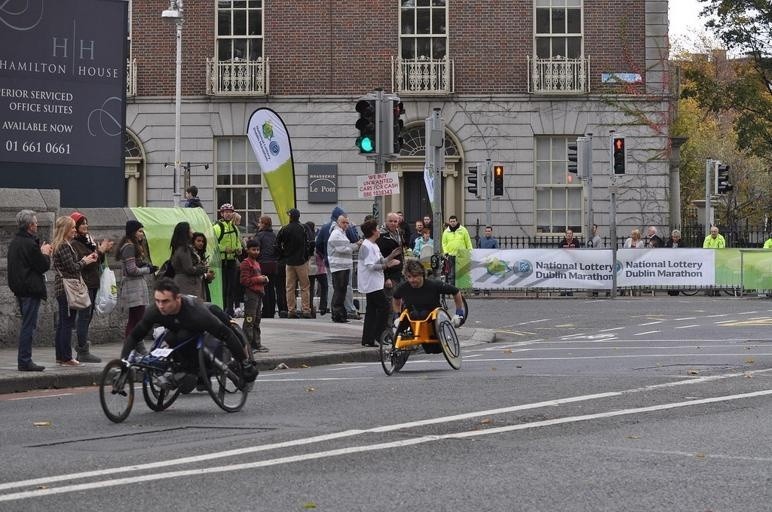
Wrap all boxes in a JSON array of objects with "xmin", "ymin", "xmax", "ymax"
[{"xmin": 219, "ymin": 203, "xmax": 234, "ymax": 212}]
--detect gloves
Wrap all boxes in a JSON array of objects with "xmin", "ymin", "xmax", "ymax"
[
  {"xmin": 450, "ymin": 314, "xmax": 464, "ymax": 328},
  {"xmin": 149, "ymin": 264, "xmax": 159, "ymax": 274},
  {"xmin": 237, "ymin": 358, "xmax": 259, "ymax": 389}
]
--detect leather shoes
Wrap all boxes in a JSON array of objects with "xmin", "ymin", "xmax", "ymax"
[
  {"xmin": 17, "ymin": 363, "xmax": 45, "ymax": 371},
  {"xmin": 278, "ymin": 308, "xmax": 362, "ymax": 323}
]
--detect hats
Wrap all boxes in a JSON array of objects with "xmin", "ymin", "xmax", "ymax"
[
  {"xmin": 71, "ymin": 212, "xmax": 85, "ymax": 222},
  {"xmin": 125, "ymin": 220, "xmax": 144, "ymax": 237},
  {"xmin": 286, "ymin": 209, "xmax": 300, "ymax": 217}
]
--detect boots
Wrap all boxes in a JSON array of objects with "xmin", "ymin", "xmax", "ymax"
[{"xmin": 75, "ymin": 341, "xmax": 101, "ymax": 363}]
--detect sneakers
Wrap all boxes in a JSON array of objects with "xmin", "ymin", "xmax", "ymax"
[
  {"xmin": 234, "ymin": 307, "xmax": 245, "ymax": 318},
  {"xmin": 257, "ymin": 345, "xmax": 269, "ymax": 352},
  {"xmin": 60, "ymin": 358, "xmax": 80, "ymax": 367},
  {"xmin": 152, "ymin": 366, "xmax": 177, "ymax": 392}
]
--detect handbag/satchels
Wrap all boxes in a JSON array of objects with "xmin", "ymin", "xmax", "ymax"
[
  {"xmin": 63, "ymin": 278, "xmax": 92, "ymax": 317},
  {"xmin": 93, "ymin": 253, "xmax": 118, "ymax": 318},
  {"xmin": 155, "ymin": 260, "xmax": 175, "ymax": 280}
]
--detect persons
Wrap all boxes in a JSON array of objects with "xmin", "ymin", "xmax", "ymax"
[
  {"xmin": 167, "ymin": 186, "xmax": 472, "ymax": 353},
  {"xmin": 472, "ymin": 226, "xmax": 499, "ymax": 296},
  {"xmin": 68, "ymin": 212, "xmax": 115, "ymax": 363},
  {"xmin": 644, "ymin": 226, "xmax": 664, "ymax": 294},
  {"xmin": 621, "ymin": 229, "xmax": 644, "ymax": 297},
  {"xmin": 49, "ymin": 216, "xmax": 98, "ymax": 368},
  {"xmin": 702, "ymin": 227, "xmax": 726, "ymax": 296},
  {"xmin": 7, "ymin": 209, "xmax": 54, "ymax": 371},
  {"xmin": 114, "ymin": 278, "xmax": 258, "ymax": 383},
  {"xmin": 762, "ymin": 232, "xmax": 772, "ymax": 296},
  {"xmin": 665, "ymin": 229, "xmax": 684, "ymax": 296},
  {"xmin": 114, "ymin": 219, "xmax": 159, "ymax": 342},
  {"xmin": 558, "ymin": 230, "xmax": 580, "ymax": 295},
  {"xmin": 587, "ymin": 224, "xmax": 601, "ymax": 298}
]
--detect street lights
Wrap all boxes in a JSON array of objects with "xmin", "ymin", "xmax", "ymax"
[
  {"xmin": 159, "ymin": 0, "xmax": 189, "ymax": 208},
  {"xmin": 162, "ymin": 162, "xmax": 209, "ymax": 200}
]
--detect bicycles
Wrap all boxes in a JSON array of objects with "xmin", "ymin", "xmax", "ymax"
[{"xmin": 680, "ymin": 289, "xmax": 741, "ymax": 296}]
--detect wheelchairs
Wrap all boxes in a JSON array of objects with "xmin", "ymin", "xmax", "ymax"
[
  {"xmin": 375, "ymin": 293, "xmax": 470, "ymax": 375},
  {"xmin": 97, "ymin": 309, "xmax": 255, "ymax": 426}
]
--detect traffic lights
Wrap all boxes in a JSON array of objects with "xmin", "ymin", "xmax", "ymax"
[
  {"xmin": 354, "ymin": 93, "xmax": 381, "ymax": 158},
  {"xmin": 613, "ymin": 137, "xmax": 626, "ymax": 176},
  {"xmin": 722, "ymin": 180, "xmax": 733, "ymax": 197},
  {"xmin": 492, "ymin": 165, "xmax": 504, "ymax": 199},
  {"xmin": 382, "ymin": 96, "xmax": 406, "ymax": 155},
  {"xmin": 714, "ymin": 161, "xmax": 729, "ymax": 195},
  {"xmin": 567, "ymin": 137, "xmax": 588, "ymax": 178},
  {"xmin": 466, "ymin": 162, "xmax": 482, "ymax": 199}
]
